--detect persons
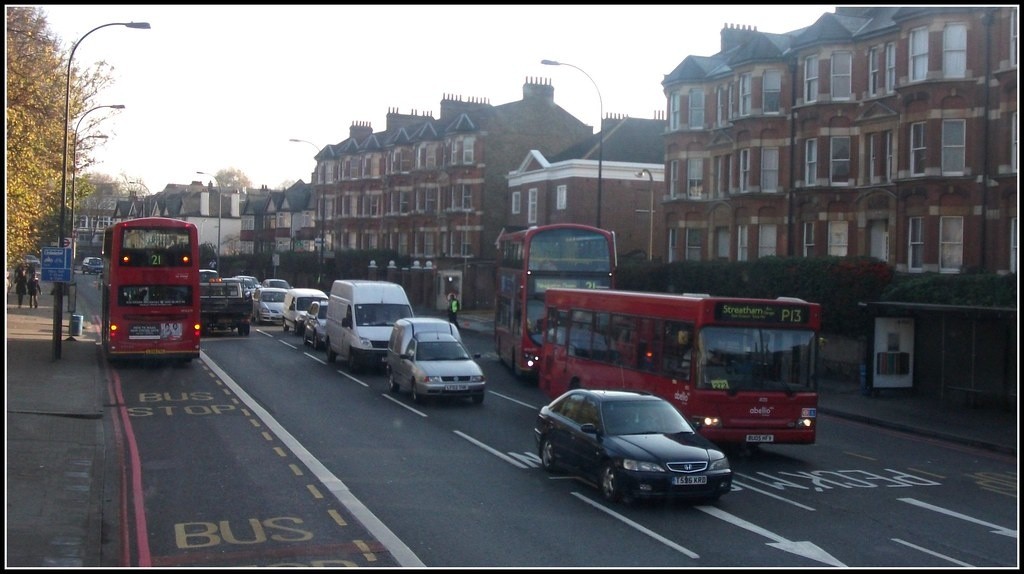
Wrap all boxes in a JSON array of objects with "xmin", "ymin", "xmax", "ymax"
[
  {"xmin": 447, "ymin": 287, "xmax": 461, "ymax": 330},
  {"xmin": 13, "ymin": 260, "xmax": 42, "ymax": 310}
]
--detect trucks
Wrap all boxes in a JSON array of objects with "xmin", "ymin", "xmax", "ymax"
[{"xmin": 197, "ymin": 279, "xmax": 255, "ymax": 337}]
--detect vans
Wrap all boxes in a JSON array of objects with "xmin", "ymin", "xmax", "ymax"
[
  {"xmin": 281, "ymin": 289, "xmax": 328, "ymax": 332},
  {"xmin": 326, "ymin": 278, "xmax": 417, "ymax": 371}
]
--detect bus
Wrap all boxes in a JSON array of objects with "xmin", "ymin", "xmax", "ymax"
[
  {"xmin": 491, "ymin": 224, "xmax": 619, "ymax": 378},
  {"xmin": 100, "ymin": 217, "xmax": 203, "ymax": 365},
  {"xmin": 538, "ymin": 284, "xmax": 826, "ymax": 457}
]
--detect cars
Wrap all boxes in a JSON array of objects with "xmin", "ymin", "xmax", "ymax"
[
  {"xmin": 534, "ymin": 387, "xmax": 732, "ymax": 505},
  {"xmin": 24, "ymin": 255, "xmax": 41, "ymax": 266}
]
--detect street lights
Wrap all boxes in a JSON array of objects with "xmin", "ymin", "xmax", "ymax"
[
  {"xmin": 65, "ymin": 133, "xmax": 108, "ymax": 256},
  {"xmin": 129, "ymin": 180, "xmax": 153, "ymax": 217},
  {"xmin": 196, "ymin": 171, "xmax": 221, "ymax": 276},
  {"xmin": 635, "ymin": 168, "xmax": 655, "ymax": 266},
  {"xmin": 541, "ymin": 58, "xmax": 605, "ymax": 228},
  {"xmin": 68, "ymin": 104, "xmax": 125, "ymax": 298},
  {"xmin": 52, "ymin": 21, "xmax": 151, "ymax": 357},
  {"xmin": 289, "ymin": 138, "xmax": 328, "ymax": 290}
]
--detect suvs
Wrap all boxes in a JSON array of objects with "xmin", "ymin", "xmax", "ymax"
[
  {"xmin": 197, "ymin": 268, "xmax": 293, "ymax": 324},
  {"xmin": 386, "ymin": 318, "xmax": 487, "ymax": 406},
  {"xmin": 81, "ymin": 257, "xmax": 105, "ymax": 275},
  {"xmin": 304, "ymin": 300, "xmax": 333, "ymax": 348}
]
